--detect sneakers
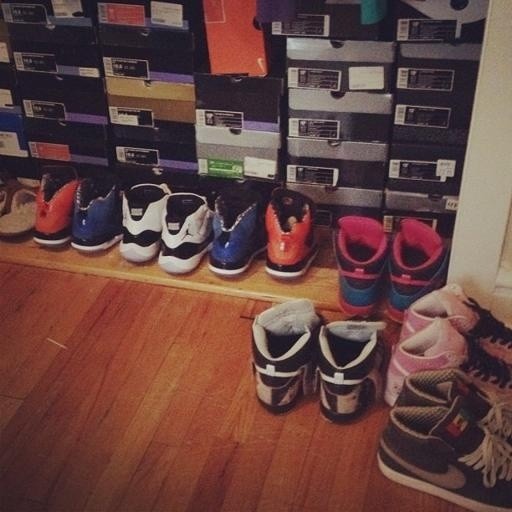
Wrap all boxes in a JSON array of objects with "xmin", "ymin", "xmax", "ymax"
[{"xmin": 251, "ymin": 298, "xmax": 324, "ymax": 414}]
